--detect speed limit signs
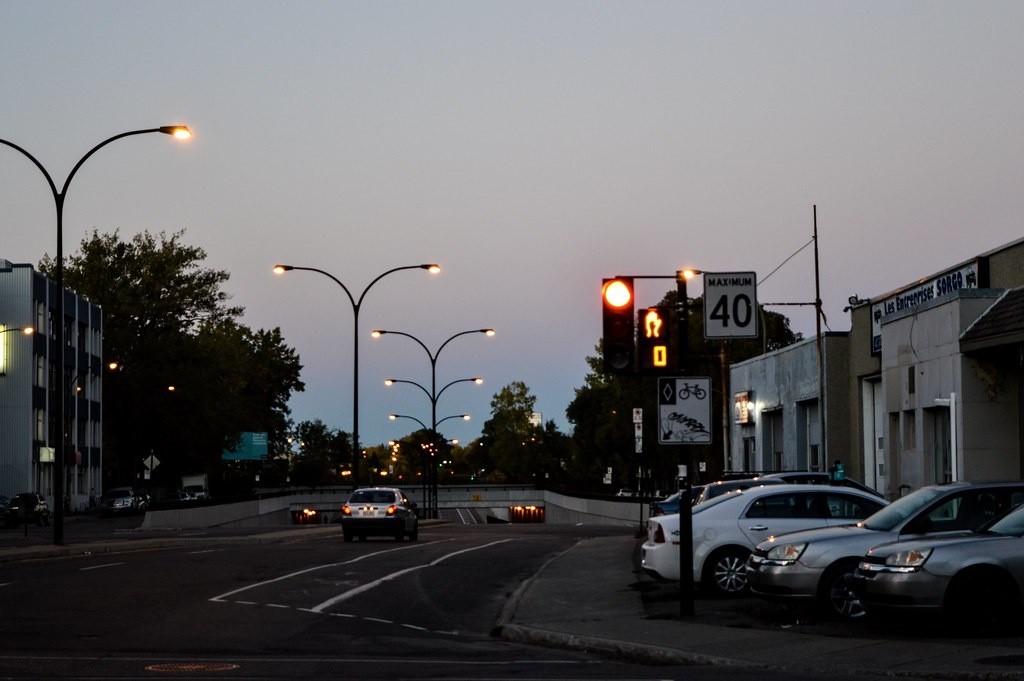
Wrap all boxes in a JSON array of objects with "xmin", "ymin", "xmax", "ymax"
[{"xmin": 704, "ymin": 271, "xmax": 759, "ymax": 340}]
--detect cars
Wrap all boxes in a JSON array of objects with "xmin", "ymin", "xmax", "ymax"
[
  {"xmin": 652, "ymin": 468, "xmax": 888, "ymax": 519},
  {"xmin": 1, "ymin": 492, "xmax": 50, "ymax": 526},
  {"xmin": 746, "ymin": 478, "xmax": 1023, "ymax": 631},
  {"xmin": 640, "ymin": 483, "xmax": 892, "ymax": 604},
  {"xmin": 107, "ymin": 488, "xmax": 134, "ymax": 513},
  {"xmin": 849, "ymin": 493, "xmax": 1024, "ymax": 637},
  {"xmin": 342, "ymin": 486, "xmax": 417, "ymax": 542}
]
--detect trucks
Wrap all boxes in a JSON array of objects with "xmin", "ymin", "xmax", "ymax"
[{"xmin": 181, "ymin": 473, "xmax": 209, "ymax": 502}]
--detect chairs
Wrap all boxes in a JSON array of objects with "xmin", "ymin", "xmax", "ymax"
[
  {"xmin": 807, "ymin": 497, "xmax": 832, "ymax": 520},
  {"xmin": 950, "ymin": 498, "xmax": 981, "ymax": 527}
]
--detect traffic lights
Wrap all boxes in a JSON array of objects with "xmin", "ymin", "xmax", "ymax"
[
  {"xmin": 637, "ymin": 308, "xmax": 674, "ymax": 380},
  {"xmin": 600, "ymin": 276, "xmax": 636, "ymax": 378}
]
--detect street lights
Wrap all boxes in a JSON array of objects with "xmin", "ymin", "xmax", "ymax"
[
  {"xmin": 277, "ymin": 261, "xmax": 440, "ymax": 492},
  {"xmin": 371, "ymin": 326, "xmax": 496, "ymax": 519},
  {"xmin": 0, "ymin": 120, "xmax": 183, "ymax": 546}
]
